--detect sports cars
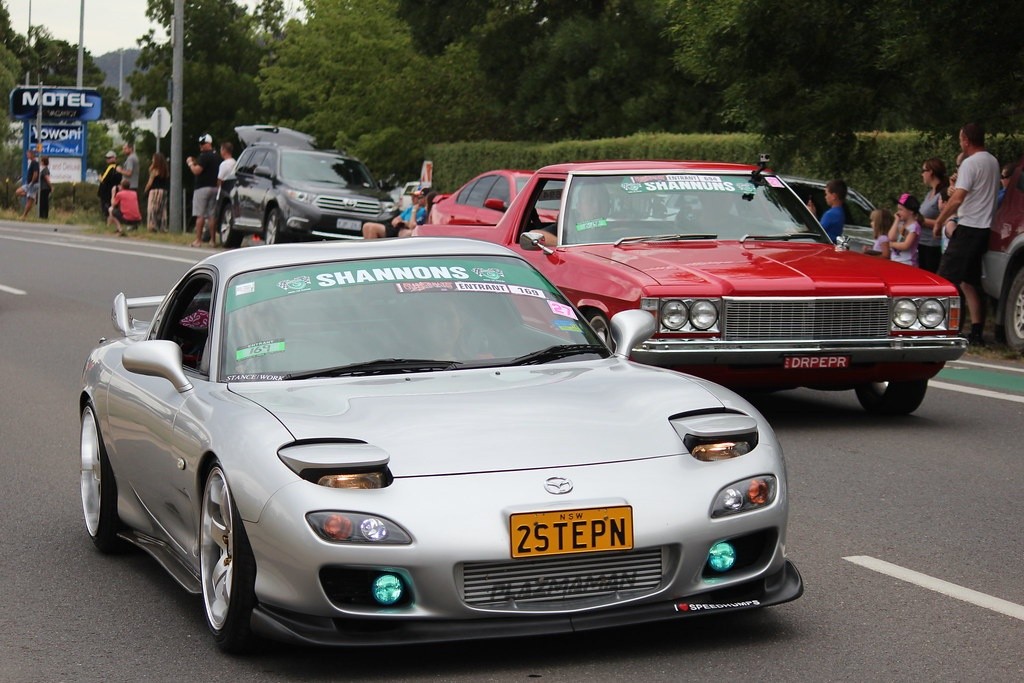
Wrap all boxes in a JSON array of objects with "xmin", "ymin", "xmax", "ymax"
[{"xmin": 76, "ymin": 236, "xmax": 806, "ymax": 652}]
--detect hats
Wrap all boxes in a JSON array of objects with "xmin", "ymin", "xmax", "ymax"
[
  {"xmin": 899, "ymin": 194, "xmax": 920, "ymax": 211},
  {"xmin": 103, "ymin": 150, "xmax": 116, "ymax": 159},
  {"xmin": 198, "ymin": 134, "xmax": 212, "ymax": 143},
  {"xmin": 406, "ymin": 186, "xmax": 418, "ymax": 195}
]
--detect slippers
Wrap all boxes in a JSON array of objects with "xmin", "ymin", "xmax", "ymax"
[{"xmin": 188, "ymin": 243, "xmax": 201, "ymax": 248}]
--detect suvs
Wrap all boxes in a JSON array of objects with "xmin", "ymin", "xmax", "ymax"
[{"xmin": 217, "ymin": 123, "xmax": 403, "ymax": 245}]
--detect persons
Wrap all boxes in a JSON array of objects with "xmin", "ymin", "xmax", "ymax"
[
  {"xmin": 805, "ymin": 120, "xmax": 1024, "ymax": 348},
  {"xmin": 528, "ymin": 182, "xmax": 618, "ymax": 246},
  {"xmin": 361, "ymin": 184, "xmax": 436, "ymax": 240},
  {"xmin": 97, "ymin": 140, "xmax": 142, "ymax": 239},
  {"xmin": 143, "ymin": 152, "xmax": 170, "ymax": 233},
  {"xmin": 16, "ymin": 150, "xmax": 55, "ymax": 222},
  {"xmin": 411, "ymin": 295, "xmax": 475, "ymax": 362},
  {"xmin": 186, "ymin": 132, "xmax": 237, "ymax": 250}
]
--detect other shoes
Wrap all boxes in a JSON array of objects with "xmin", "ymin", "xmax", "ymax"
[
  {"xmin": 955, "ymin": 334, "xmax": 985, "ymax": 347},
  {"xmin": 126, "ymin": 224, "xmax": 138, "ymax": 232},
  {"xmin": 116, "ymin": 230, "xmax": 125, "ymax": 237}
]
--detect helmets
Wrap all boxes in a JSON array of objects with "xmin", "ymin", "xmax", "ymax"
[{"xmin": 415, "ymin": 185, "xmax": 432, "ymax": 196}]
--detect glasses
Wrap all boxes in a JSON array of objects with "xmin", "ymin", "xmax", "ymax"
[
  {"xmin": 1000, "ymin": 174, "xmax": 1009, "ymax": 179},
  {"xmin": 956, "ymin": 164, "xmax": 961, "ymax": 168},
  {"xmin": 921, "ymin": 168, "xmax": 930, "ymax": 173}
]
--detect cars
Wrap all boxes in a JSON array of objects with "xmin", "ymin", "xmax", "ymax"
[{"xmin": 416, "ymin": 151, "xmax": 1023, "ymax": 424}]
